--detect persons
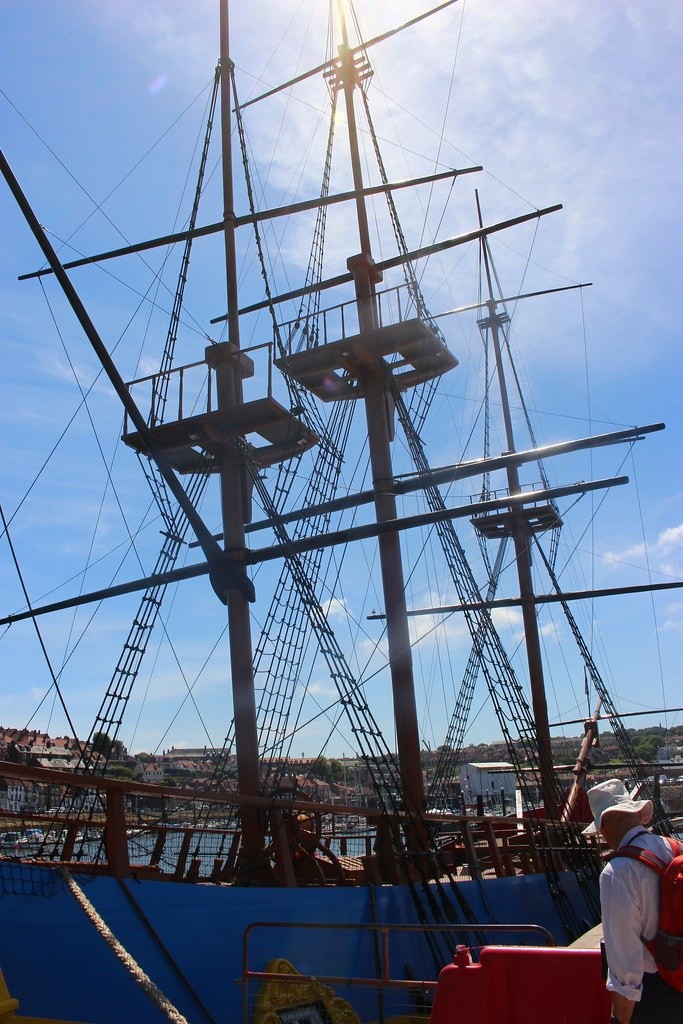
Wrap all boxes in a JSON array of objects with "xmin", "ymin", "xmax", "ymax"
[{"xmin": 581, "ymin": 779, "xmax": 683, "ymax": 1024}]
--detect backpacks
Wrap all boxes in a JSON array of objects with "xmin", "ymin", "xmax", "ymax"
[{"xmin": 604, "ymin": 837, "xmax": 683, "ymax": 993}]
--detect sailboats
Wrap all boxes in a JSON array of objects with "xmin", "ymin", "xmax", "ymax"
[{"xmin": 0, "ymin": 2, "xmax": 683, "ymax": 1023}]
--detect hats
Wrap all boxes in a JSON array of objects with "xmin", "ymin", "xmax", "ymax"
[{"xmin": 581, "ymin": 779, "xmax": 653, "ymax": 835}]
